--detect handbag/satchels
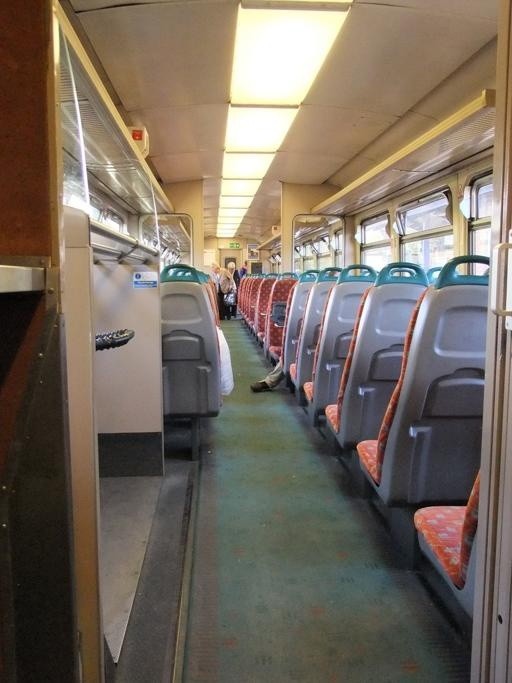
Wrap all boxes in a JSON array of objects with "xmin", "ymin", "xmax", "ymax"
[{"xmin": 223, "ymin": 289, "xmax": 236, "ymax": 306}]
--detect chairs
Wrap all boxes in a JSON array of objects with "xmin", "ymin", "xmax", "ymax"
[
  {"xmin": 355, "ymin": 253, "xmax": 492, "ymax": 506},
  {"xmin": 161, "ymin": 263, "xmax": 226, "ymax": 461},
  {"xmin": 324, "ymin": 261, "xmax": 429, "ymax": 452},
  {"xmin": 237, "ymin": 253, "xmax": 494, "ymax": 427},
  {"xmin": 412, "ymin": 466, "xmax": 480, "ymax": 622}
]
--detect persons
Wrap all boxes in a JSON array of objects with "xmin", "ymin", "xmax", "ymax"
[
  {"xmin": 227, "ymin": 262, "xmax": 240, "ymax": 316},
  {"xmin": 251, "ymin": 362, "xmax": 283, "ymax": 391},
  {"xmin": 238, "ymin": 262, "xmax": 248, "ymax": 277},
  {"xmin": 213, "ymin": 263, "xmax": 237, "ymax": 319}
]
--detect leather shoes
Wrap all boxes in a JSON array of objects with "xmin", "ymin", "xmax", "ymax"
[{"xmin": 251, "ymin": 382, "xmax": 269, "ymax": 392}]
